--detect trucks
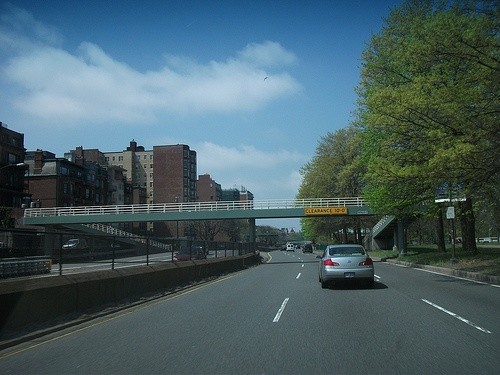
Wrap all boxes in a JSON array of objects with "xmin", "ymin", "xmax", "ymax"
[{"xmin": 286, "ymin": 241, "xmax": 295, "ymax": 252}]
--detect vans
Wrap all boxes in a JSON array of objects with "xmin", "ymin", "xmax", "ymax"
[{"xmin": 302, "ymin": 243, "xmax": 313, "ymax": 254}]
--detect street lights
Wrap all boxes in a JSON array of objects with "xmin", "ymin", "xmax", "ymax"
[{"xmin": 174, "ymin": 196, "xmax": 180, "ymax": 239}]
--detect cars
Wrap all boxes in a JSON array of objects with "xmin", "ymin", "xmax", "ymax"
[
  {"xmin": 62, "ymin": 239, "xmax": 88, "ymax": 249},
  {"xmin": 316, "ymin": 244, "xmax": 374, "ymax": 288},
  {"xmin": 173, "ymin": 240, "xmax": 209, "ymax": 263}
]
